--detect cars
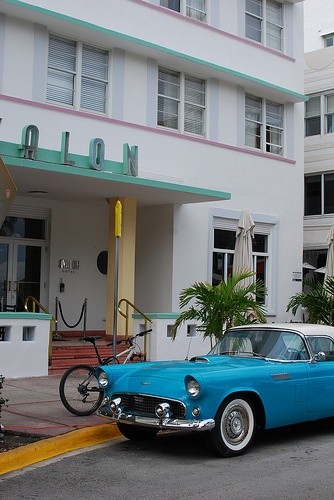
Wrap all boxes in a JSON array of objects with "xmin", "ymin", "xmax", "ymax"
[{"xmin": 95, "ymin": 324, "xmax": 334, "ymax": 457}]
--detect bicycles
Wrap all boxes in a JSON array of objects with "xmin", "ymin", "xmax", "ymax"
[{"xmin": 59, "ymin": 328, "xmax": 154, "ymax": 416}]
